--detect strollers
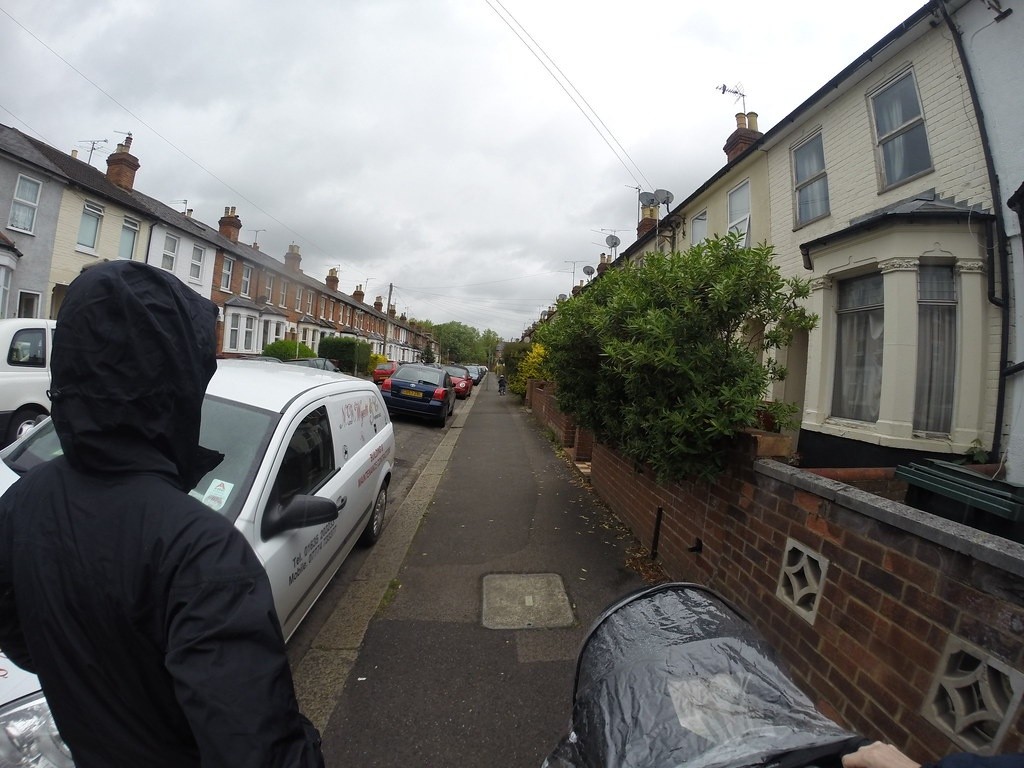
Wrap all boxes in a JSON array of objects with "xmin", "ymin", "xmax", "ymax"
[{"xmin": 541, "ymin": 582, "xmax": 880, "ymax": 768}]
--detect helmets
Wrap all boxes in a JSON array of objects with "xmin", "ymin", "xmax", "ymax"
[{"xmin": 500, "ymin": 375, "xmax": 504, "ymax": 377}]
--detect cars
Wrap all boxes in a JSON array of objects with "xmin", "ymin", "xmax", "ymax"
[
  {"xmin": 0, "ymin": 318, "xmax": 57, "ymax": 441},
  {"xmin": 0, "ymin": 353, "xmax": 396, "ymax": 768},
  {"xmin": 443, "ymin": 365, "xmax": 472, "ymax": 400},
  {"xmin": 478, "ymin": 367, "xmax": 484, "ymax": 379},
  {"xmin": 239, "ymin": 355, "xmax": 283, "ymax": 365},
  {"xmin": 464, "ymin": 366, "xmax": 481, "ymax": 386},
  {"xmin": 481, "ymin": 367, "xmax": 486, "ymax": 375},
  {"xmin": 380, "ymin": 364, "xmax": 457, "ymax": 428},
  {"xmin": 426, "ymin": 362, "xmax": 442, "ymax": 369},
  {"xmin": 373, "ymin": 359, "xmax": 398, "ymax": 384},
  {"xmin": 283, "ymin": 357, "xmax": 343, "ymax": 374}
]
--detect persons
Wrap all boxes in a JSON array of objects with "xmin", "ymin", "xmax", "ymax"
[
  {"xmin": 842, "ymin": 741, "xmax": 1024, "ymax": 768},
  {"xmin": 0, "ymin": 261, "xmax": 326, "ymax": 768},
  {"xmin": 498, "ymin": 375, "xmax": 507, "ymax": 393}
]
